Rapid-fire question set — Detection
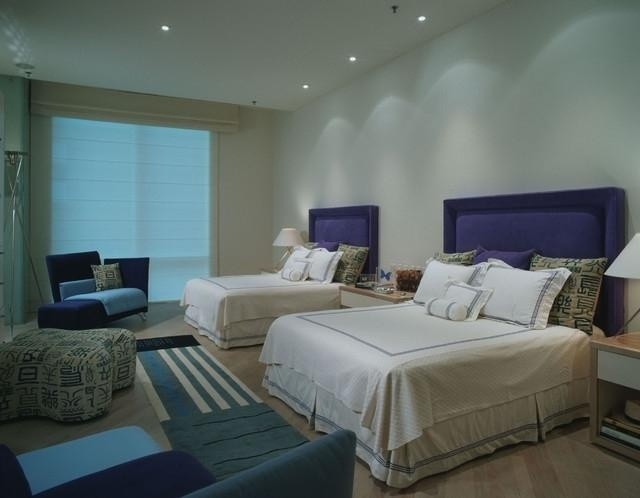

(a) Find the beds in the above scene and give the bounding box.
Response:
[271,187,625,489]
[186,205,379,350]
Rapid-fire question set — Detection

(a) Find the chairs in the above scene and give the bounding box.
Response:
[37,251,150,328]
[0,425,356,498]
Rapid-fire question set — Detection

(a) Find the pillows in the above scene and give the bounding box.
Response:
[90,262,124,292]
[278,240,370,285]
[412,247,609,336]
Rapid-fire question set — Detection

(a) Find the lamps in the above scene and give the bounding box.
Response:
[603,233,640,279]
[271,228,304,257]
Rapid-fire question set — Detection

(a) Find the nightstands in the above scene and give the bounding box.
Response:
[340,283,415,310]
[260,269,275,275]
[589,332,640,464]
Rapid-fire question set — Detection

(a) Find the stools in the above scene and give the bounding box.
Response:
[0,328,137,423]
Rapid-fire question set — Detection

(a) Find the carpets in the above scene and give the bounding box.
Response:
[136,335,312,484]
